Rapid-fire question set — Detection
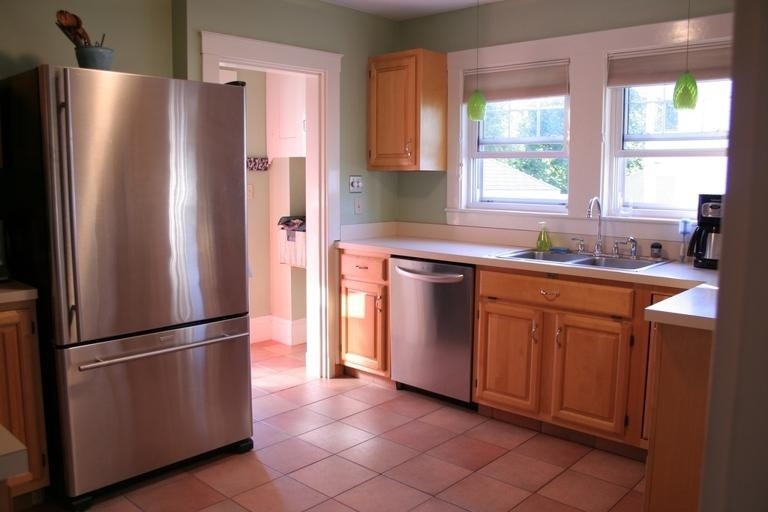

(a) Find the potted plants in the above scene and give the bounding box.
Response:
[52,9,115,71]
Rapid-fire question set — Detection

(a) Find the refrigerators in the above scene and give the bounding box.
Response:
[1,65,253,501]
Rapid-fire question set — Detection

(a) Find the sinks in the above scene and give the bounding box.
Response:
[573,252,674,273]
[497,248,587,268]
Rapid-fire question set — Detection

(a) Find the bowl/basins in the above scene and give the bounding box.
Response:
[73,47,115,73]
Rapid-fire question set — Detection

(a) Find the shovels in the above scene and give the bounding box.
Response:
[56,10,84,48]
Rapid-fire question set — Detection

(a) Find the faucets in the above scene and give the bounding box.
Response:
[628,237,638,260]
[586,196,604,258]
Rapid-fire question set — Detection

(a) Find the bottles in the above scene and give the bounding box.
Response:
[650,242,662,258]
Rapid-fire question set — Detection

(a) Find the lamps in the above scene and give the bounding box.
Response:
[670,0,700,110]
[465,0,489,121]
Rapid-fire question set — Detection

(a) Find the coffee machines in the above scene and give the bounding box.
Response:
[686,194,725,270]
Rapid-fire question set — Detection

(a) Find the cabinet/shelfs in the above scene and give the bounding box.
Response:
[0,298,51,511]
[625,282,678,450]
[364,45,444,173]
[337,249,397,390]
[470,264,635,457]
[265,69,307,157]
[641,321,713,512]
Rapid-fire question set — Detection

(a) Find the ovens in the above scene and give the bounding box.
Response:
[387,256,477,412]
